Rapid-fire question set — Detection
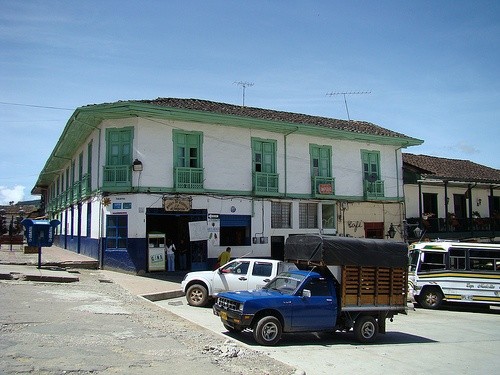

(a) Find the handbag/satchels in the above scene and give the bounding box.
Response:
[172,244,176,252]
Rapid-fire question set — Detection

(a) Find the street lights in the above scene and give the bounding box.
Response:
[389,223,405,239]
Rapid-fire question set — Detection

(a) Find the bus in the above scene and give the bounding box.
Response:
[403,242,499,308]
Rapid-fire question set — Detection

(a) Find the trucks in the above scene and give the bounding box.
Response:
[213,234,413,345]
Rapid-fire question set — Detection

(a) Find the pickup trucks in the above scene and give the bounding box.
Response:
[181,257,282,308]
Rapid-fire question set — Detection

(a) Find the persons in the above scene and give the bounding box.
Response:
[165,239,176,272]
[177,239,188,270]
[218,247,231,266]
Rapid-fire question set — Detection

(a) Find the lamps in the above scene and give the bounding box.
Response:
[388,222,401,239]
[413,227,423,238]
[133,158,143,171]
